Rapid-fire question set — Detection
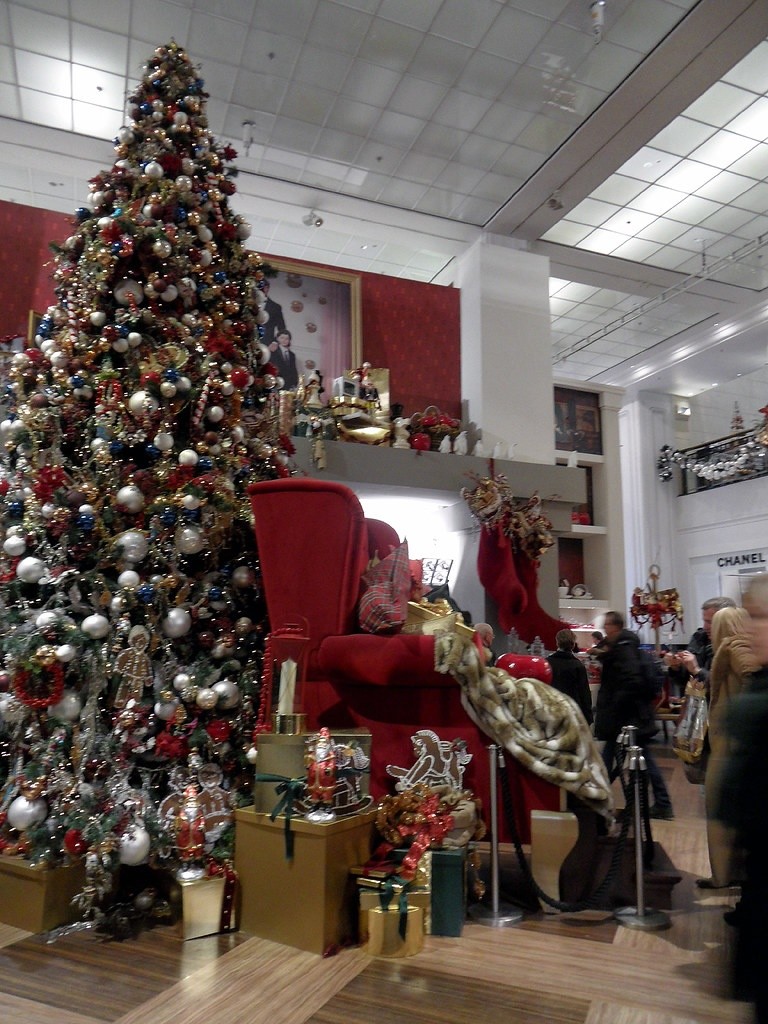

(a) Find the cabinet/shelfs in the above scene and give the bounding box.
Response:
[554,446,615,608]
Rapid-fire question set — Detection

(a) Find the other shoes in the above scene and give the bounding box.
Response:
[648,803,674,820]
[695,878,731,890]
[613,809,635,823]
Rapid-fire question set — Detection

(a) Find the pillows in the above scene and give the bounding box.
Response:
[400,612,458,634]
[356,536,411,634]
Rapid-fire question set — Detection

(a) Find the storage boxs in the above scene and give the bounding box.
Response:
[350,810,578,958]
[0,852,86,933]
[578,651,604,683]
[405,600,476,643]
[256,729,372,814]
[233,804,381,957]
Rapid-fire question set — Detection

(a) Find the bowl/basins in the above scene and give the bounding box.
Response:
[558,587,568,596]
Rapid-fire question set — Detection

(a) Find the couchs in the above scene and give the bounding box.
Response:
[245,480,559,860]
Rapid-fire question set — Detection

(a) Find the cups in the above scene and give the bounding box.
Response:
[275,714,307,736]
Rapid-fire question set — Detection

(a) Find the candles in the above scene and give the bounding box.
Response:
[278,658,296,713]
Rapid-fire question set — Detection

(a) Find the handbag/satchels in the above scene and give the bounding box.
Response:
[683,731,712,784]
[673,678,708,763]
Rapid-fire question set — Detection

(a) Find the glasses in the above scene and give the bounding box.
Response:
[486,631,496,638]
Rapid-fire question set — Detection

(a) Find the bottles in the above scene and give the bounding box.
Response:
[530,636,546,658]
[507,628,519,656]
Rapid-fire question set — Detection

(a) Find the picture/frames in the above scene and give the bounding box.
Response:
[256,255,362,407]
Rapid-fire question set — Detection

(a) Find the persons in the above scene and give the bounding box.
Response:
[176,789,205,871]
[260,280,299,391]
[545,629,594,727]
[306,727,336,813]
[588,612,675,824]
[473,623,497,667]
[664,573,768,1024]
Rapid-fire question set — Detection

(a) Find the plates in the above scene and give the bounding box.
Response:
[559,595,572,599]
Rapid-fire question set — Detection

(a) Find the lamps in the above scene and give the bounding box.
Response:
[302,207,323,227]
